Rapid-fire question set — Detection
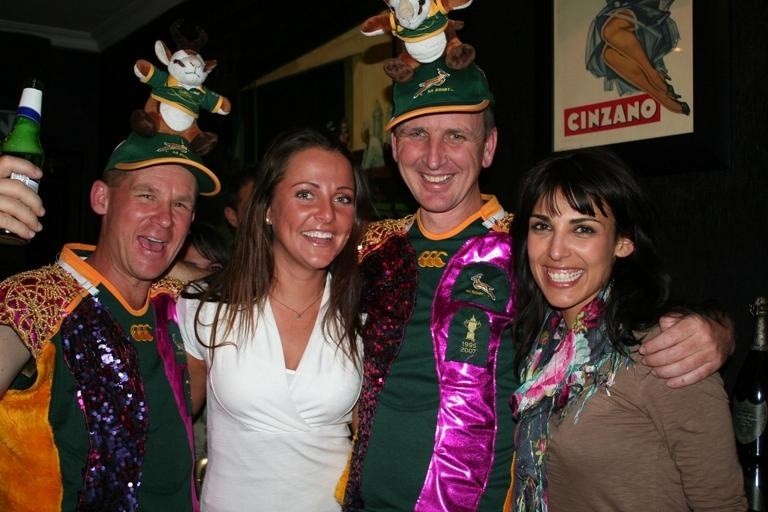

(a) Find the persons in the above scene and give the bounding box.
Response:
[0,130,204,512]
[198,151,258,230]
[166,50,739,511]
[582,1,692,115]
[503,145,752,511]
[174,125,367,512]
[177,207,238,273]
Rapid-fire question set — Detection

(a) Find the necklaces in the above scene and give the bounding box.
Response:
[265,283,325,323]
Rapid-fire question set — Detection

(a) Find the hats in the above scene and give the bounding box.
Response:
[386,58,492,130]
[102,133,221,198]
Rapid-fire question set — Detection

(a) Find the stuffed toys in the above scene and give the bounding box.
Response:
[359,0,480,85]
[128,39,235,160]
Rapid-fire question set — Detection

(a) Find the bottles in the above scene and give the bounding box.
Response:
[1,74,47,246]
[731,294,766,512]
